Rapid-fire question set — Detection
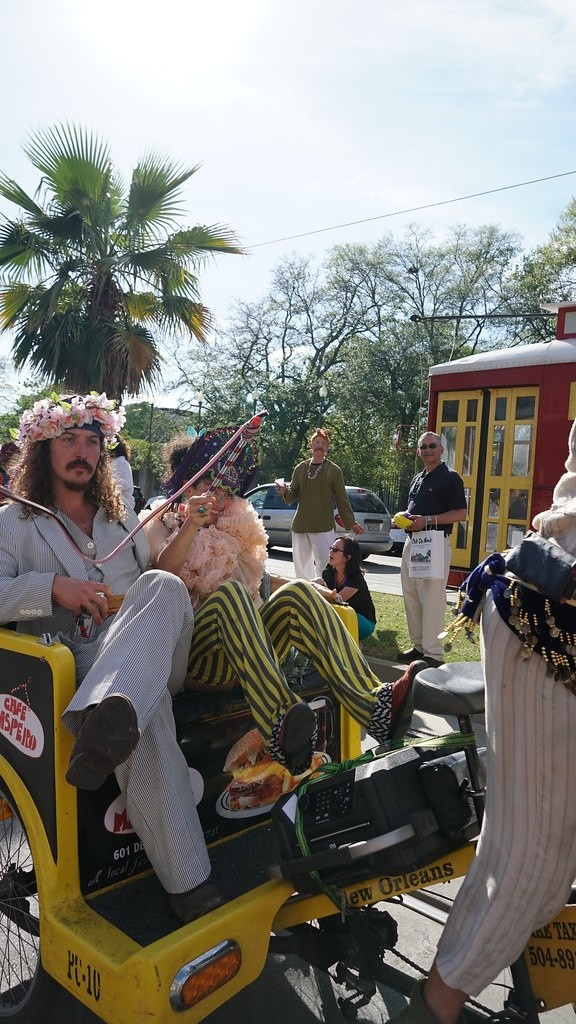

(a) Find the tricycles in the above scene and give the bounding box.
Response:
[0,570,576,1023]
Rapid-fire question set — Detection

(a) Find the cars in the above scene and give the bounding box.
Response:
[133,486,148,515]
[383,517,408,555]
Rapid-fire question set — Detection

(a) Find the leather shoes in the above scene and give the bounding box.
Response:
[65,692,140,792]
[168,878,228,924]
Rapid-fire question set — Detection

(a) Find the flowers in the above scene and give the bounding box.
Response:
[9,390,129,452]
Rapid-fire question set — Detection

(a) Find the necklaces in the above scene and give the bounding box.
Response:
[409,461,443,501]
[335,576,345,587]
[307,456,325,479]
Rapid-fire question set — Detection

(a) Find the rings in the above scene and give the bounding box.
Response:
[197,504,206,513]
[201,513,202,517]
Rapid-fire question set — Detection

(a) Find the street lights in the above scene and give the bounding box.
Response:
[196,393,205,439]
[147,393,156,444]
[316,384,329,429]
[252,387,259,416]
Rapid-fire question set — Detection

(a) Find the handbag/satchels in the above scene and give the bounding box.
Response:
[408,514,445,578]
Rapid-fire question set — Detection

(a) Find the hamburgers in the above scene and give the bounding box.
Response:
[223,726,324,809]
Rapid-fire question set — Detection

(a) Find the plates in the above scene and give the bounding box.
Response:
[216,750,332,819]
[104,767,204,835]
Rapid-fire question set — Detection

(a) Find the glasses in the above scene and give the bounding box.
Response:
[329,546,344,552]
[417,443,438,450]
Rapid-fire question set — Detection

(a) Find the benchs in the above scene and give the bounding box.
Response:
[0,580,366,899]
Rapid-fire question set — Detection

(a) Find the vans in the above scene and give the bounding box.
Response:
[244,482,394,561]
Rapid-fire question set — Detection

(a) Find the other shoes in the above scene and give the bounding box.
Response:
[387,659,429,740]
[397,647,424,660]
[420,656,445,669]
[278,702,317,776]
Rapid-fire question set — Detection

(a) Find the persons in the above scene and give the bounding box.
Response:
[392,432,467,668]
[0,390,230,927]
[138,437,431,775]
[322,537,377,639]
[274,432,364,580]
[106,433,135,510]
[0,441,21,506]
[384,417,576,1024]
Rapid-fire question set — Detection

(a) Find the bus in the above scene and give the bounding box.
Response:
[394,299,575,589]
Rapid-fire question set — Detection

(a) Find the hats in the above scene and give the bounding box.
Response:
[0,441,20,465]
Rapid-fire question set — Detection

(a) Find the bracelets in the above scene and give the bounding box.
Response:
[427,515,433,525]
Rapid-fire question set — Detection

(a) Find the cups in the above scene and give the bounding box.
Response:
[275,478,284,491]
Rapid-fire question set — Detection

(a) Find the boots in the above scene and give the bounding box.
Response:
[383,978,467,1024]
